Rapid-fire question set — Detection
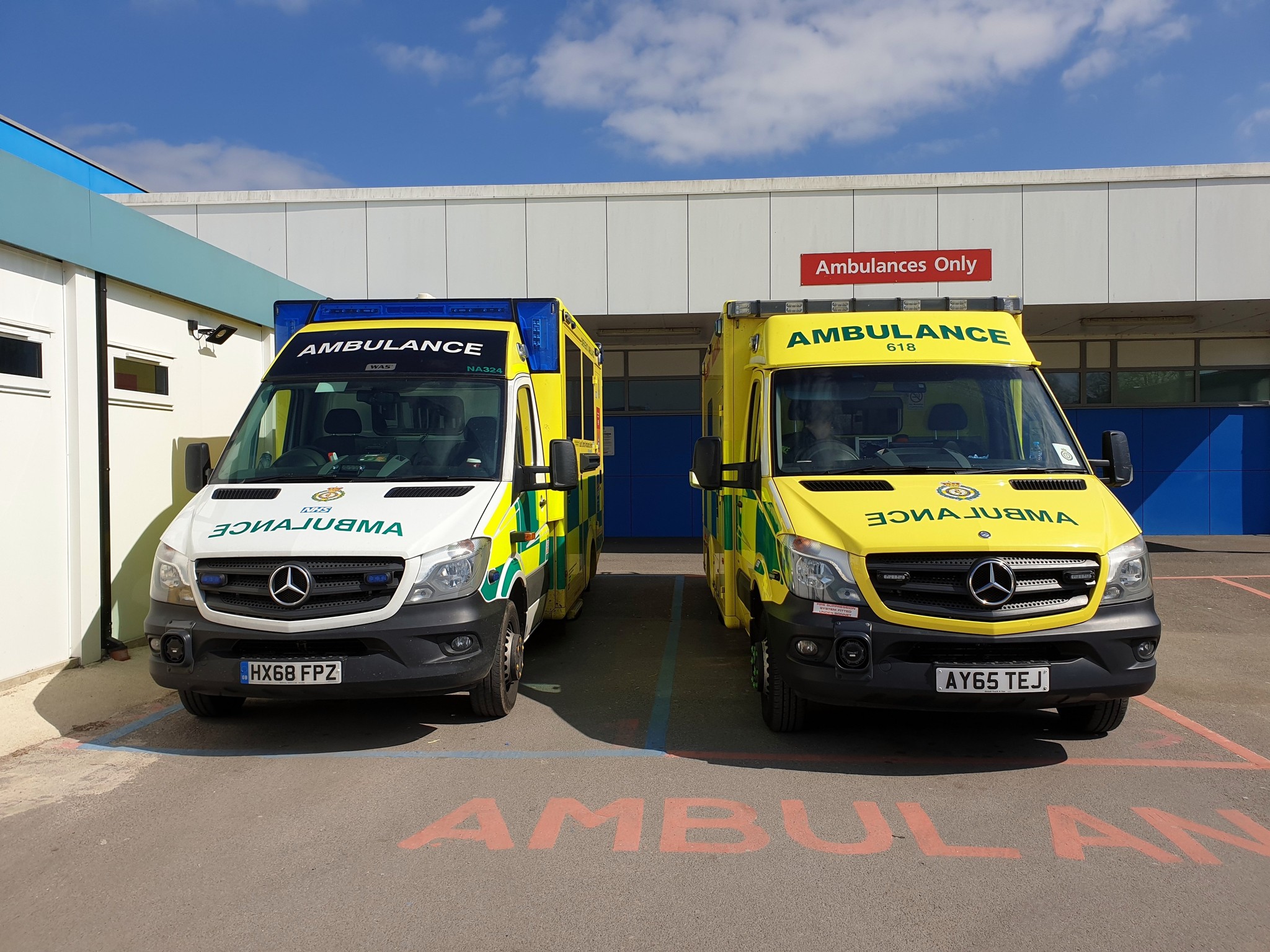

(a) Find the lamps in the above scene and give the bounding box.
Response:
[188,320,238,345]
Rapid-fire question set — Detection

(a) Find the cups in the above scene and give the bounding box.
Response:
[467,459,481,468]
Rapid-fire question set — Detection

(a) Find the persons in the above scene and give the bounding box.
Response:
[781,406,835,464]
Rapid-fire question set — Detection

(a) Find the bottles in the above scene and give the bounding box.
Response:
[257,451,272,467]
[1030,442,1042,461]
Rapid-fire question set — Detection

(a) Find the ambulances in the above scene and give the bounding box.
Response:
[142,291,607,724]
[687,297,1164,740]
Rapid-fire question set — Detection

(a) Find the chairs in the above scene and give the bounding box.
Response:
[788,398,829,434]
[312,408,366,456]
[447,416,498,467]
[929,403,968,440]
